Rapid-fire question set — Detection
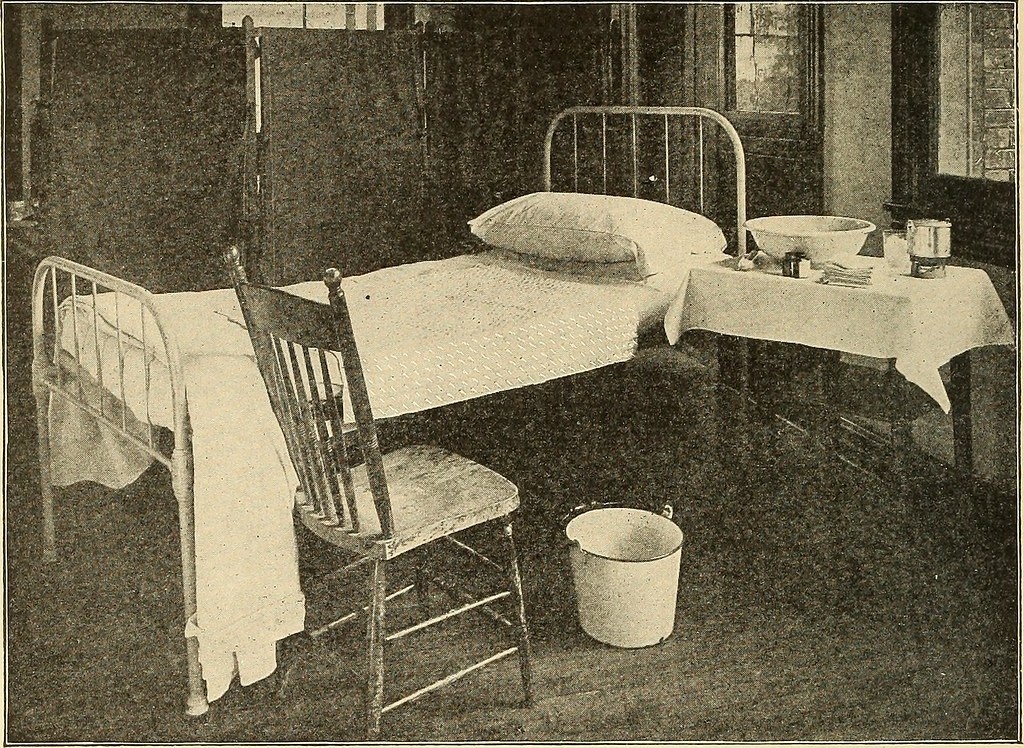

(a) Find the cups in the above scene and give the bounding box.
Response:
[882,229,911,275]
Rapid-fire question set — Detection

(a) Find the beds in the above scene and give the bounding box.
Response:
[31,106,747,717]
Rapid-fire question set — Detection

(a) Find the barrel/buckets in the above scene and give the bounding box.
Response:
[559,502,683,649]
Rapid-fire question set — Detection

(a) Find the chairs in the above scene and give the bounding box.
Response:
[219,244,538,742]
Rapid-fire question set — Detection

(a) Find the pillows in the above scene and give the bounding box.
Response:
[467,191,728,282]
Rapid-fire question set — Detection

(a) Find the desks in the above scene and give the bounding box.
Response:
[663,251,1018,597]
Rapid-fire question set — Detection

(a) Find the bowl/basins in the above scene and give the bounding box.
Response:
[743,215,875,263]
